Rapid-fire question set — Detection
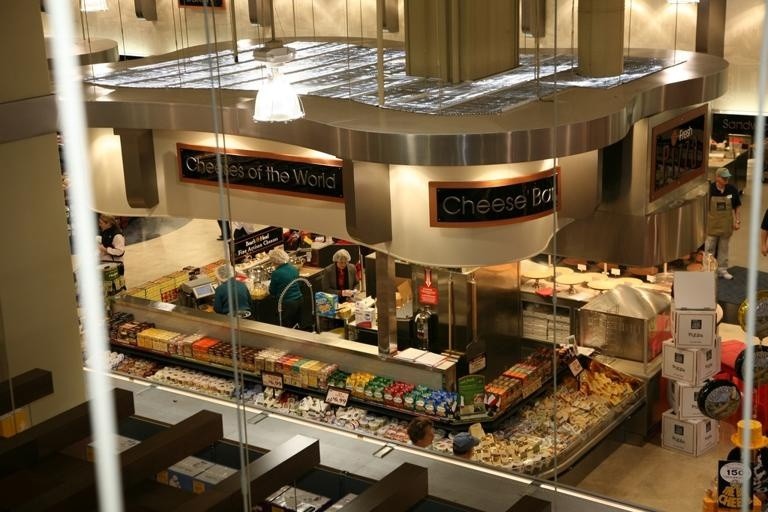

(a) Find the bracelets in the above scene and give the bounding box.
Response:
[735,221,741,224]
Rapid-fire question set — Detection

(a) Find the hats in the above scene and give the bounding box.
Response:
[452,432,481,455]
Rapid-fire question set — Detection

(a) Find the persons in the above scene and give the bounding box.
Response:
[233,222,255,241]
[704,167,743,281]
[760,207,768,257]
[266,249,305,328]
[216,219,231,242]
[452,432,482,459]
[404,416,437,451]
[282,229,300,250]
[320,248,361,303]
[213,264,253,315]
[96,214,126,265]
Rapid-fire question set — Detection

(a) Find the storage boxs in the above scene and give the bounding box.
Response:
[659,298,724,456]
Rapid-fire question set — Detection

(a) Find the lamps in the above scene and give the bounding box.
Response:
[252,1,306,124]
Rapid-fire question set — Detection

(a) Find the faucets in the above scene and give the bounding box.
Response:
[277,277,318,332]
